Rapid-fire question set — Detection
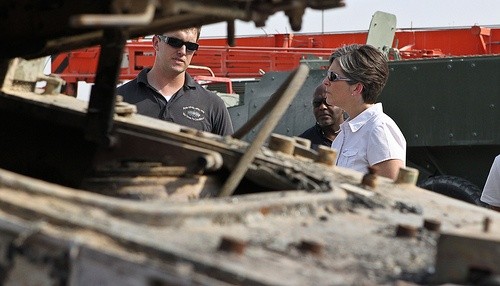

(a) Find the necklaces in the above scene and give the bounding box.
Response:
[334,127,341,134]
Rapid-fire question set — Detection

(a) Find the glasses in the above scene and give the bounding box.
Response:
[312,98,331,107]
[326,70,352,82]
[156,34,199,51]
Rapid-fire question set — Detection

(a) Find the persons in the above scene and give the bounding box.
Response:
[295,83,348,149]
[116,25,234,138]
[480,155,500,213]
[323,43,407,181]
